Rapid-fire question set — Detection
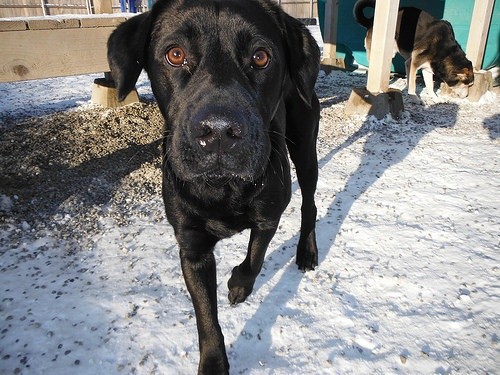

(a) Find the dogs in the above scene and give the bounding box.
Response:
[352,0,475,98]
[107,0,322,375]
